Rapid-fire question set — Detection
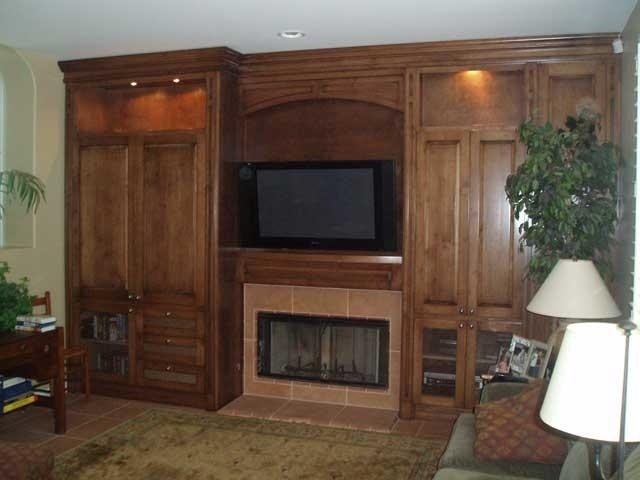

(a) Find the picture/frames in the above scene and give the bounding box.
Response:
[509,333,535,375]
[524,339,554,381]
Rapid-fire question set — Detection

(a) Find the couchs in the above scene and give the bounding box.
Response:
[432,383,590,480]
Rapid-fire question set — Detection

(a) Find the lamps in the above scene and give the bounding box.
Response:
[540,321,640,468]
[526,258,622,320]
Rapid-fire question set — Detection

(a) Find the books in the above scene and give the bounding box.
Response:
[90,311,127,343]
[15,313,56,333]
[96,349,129,378]
[0,360,70,414]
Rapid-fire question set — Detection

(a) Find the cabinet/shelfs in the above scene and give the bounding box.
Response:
[397,31,618,426]
[57,46,241,414]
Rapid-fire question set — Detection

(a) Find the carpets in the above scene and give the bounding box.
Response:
[52,407,447,480]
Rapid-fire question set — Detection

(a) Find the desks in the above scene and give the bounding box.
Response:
[0,327,66,434]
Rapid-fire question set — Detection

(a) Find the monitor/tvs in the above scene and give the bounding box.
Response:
[237,159,397,252]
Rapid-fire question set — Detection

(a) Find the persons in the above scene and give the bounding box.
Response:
[498,344,548,379]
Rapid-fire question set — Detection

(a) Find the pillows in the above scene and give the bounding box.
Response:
[473,383,568,464]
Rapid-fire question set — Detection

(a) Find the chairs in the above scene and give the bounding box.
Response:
[25,291,90,401]
